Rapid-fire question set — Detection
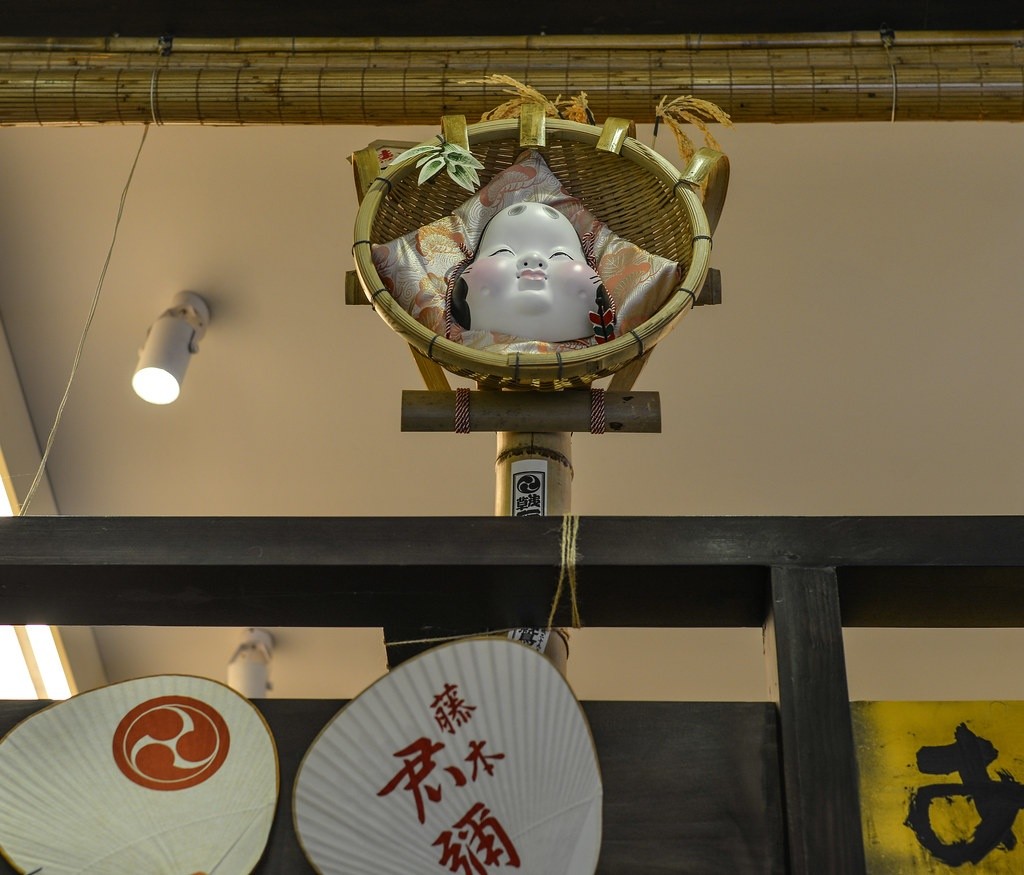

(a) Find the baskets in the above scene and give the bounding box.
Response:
[352,117,713,392]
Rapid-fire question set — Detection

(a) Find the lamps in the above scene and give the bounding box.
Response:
[228,628,277,699]
[131,293,215,405]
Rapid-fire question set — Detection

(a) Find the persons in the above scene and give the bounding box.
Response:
[451,202,617,344]
